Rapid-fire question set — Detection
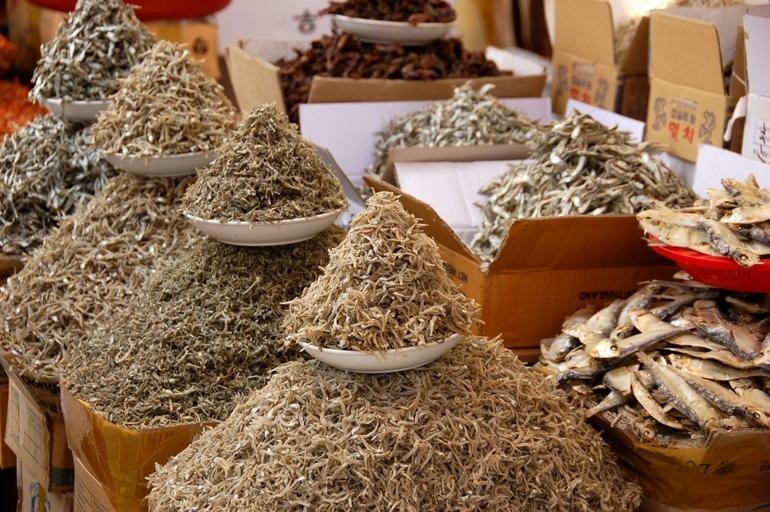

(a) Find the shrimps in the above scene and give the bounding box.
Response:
[0,0,770,512]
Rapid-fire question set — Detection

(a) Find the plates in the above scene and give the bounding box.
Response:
[297,333,465,376]
[186,208,346,248]
[329,12,457,48]
[642,222,770,293]
[103,146,222,178]
[42,96,115,121]
